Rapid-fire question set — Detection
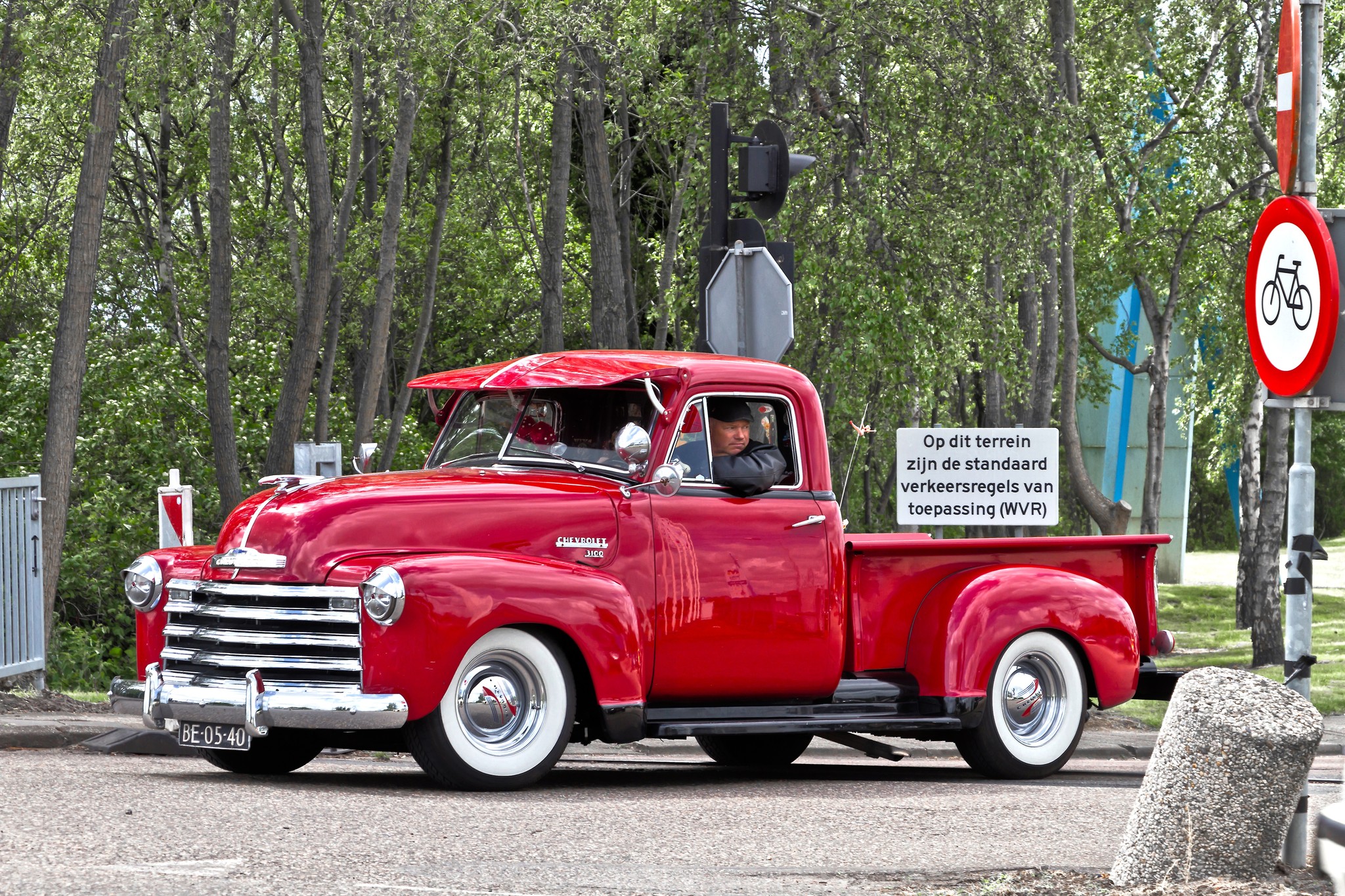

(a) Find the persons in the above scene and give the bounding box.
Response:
[670,397,789,496]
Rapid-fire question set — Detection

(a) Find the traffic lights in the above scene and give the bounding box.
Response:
[738,119,816,219]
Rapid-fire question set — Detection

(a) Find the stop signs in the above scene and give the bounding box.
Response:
[1277,0,1303,194]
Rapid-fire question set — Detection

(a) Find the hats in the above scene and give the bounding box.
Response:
[707,396,754,422]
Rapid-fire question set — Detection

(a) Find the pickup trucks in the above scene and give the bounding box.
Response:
[119,348,1179,796]
